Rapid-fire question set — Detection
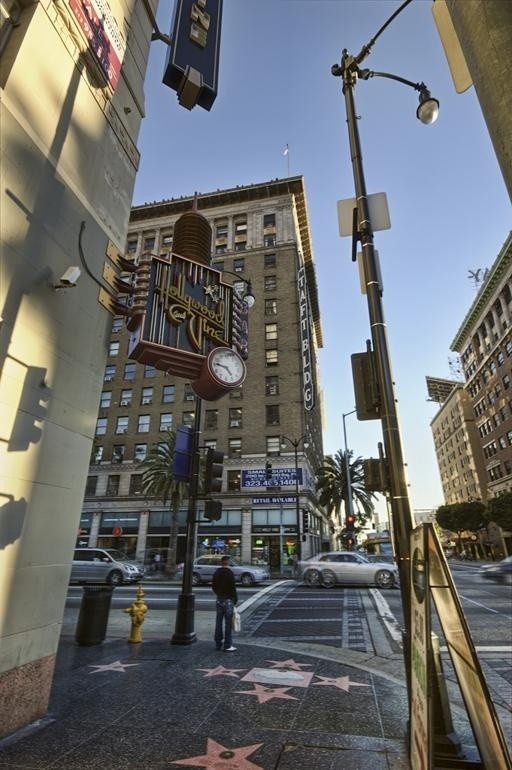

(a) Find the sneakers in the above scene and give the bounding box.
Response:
[224,645,238,653]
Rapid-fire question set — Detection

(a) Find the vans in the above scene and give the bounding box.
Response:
[134,547,176,569]
[66,546,150,586]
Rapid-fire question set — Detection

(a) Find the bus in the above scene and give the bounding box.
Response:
[361,531,394,556]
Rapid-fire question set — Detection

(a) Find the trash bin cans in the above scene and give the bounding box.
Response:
[75,586,115,646]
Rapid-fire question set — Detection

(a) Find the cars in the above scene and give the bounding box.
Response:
[366,551,395,564]
[174,552,274,587]
[476,556,512,587]
[292,550,399,590]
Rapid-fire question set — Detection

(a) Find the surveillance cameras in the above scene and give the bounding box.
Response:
[59,263,82,288]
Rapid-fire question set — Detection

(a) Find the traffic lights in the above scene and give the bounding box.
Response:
[346,532,356,546]
[346,514,355,531]
[265,460,273,481]
[303,508,310,533]
[204,444,226,494]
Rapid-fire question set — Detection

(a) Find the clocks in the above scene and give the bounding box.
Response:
[188,342,250,403]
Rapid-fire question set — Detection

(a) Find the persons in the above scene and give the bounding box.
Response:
[212,556,238,651]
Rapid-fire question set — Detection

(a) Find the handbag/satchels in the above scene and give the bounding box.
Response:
[232,606,241,633]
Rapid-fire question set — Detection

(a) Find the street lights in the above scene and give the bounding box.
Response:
[324,0,442,767]
[278,432,312,562]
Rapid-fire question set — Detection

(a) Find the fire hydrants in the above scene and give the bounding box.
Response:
[120,584,149,642]
